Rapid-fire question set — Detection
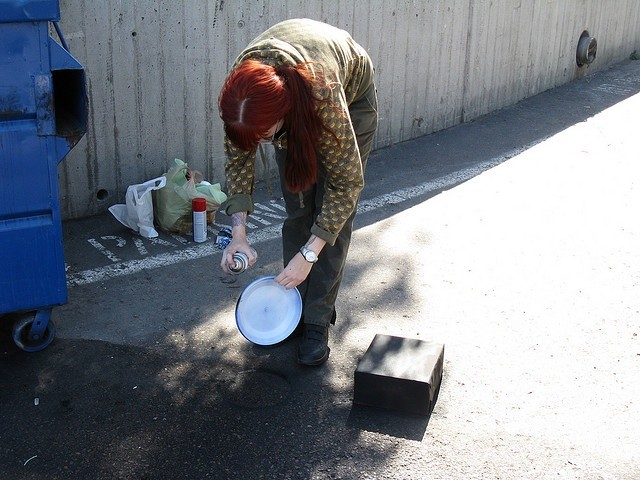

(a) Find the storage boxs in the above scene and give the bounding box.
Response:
[353,334,444,417]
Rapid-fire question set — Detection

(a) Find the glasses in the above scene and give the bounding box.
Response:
[257,124,279,145]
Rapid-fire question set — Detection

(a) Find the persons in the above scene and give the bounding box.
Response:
[218,18,379,367]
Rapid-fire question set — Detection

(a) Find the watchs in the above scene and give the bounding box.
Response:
[299,246,319,264]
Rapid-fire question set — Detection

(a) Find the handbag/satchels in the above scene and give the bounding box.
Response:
[108,175,167,238]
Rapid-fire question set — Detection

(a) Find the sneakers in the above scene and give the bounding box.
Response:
[296,320,330,366]
